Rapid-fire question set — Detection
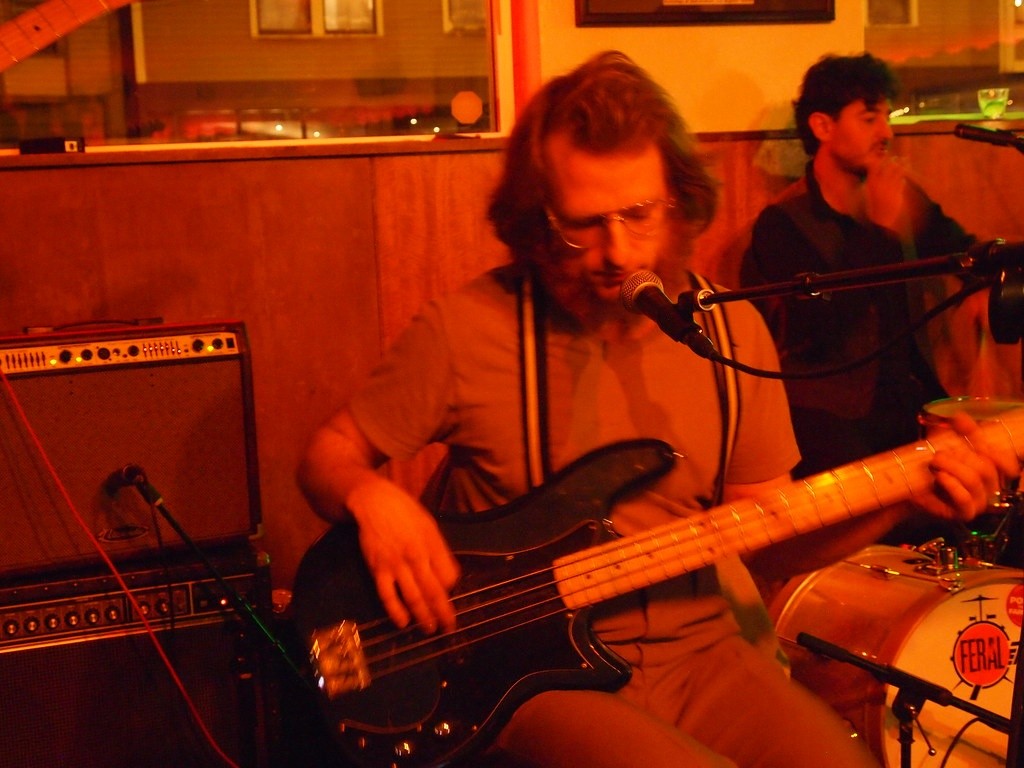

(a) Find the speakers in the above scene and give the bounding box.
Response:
[0,321,263,582]
[0,555,293,768]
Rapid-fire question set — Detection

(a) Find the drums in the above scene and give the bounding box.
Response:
[772,540,1024,767]
[918,392,1024,517]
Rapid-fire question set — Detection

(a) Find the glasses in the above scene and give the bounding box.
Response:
[539,189,678,250]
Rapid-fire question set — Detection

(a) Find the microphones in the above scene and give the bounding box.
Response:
[954,123,1024,151]
[122,463,164,508]
[620,268,719,361]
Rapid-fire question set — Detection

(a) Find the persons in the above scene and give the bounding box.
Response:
[733,50,1022,548]
[295,56,1020,768]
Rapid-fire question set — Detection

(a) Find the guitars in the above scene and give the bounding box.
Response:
[286,403,1024,768]
